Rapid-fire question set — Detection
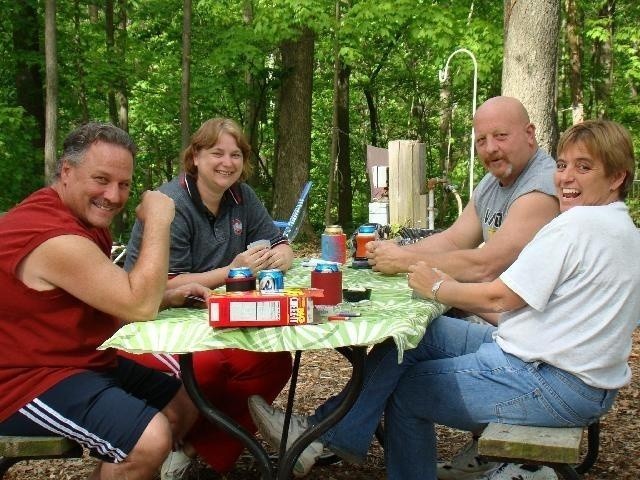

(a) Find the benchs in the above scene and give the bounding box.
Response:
[477,423,601,480]
[3,434,85,480]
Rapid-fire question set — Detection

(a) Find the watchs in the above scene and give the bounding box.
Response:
[431,278,445,303]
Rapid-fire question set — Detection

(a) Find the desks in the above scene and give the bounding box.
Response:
[94,242,450,478]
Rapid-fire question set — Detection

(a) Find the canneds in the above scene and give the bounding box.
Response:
[228,266,252,279]
[359,226,377,233]
[324,225,344,235]
[315,262,339,273]
[258,268,283,292]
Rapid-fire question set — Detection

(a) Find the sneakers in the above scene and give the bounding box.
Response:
[436,440,558,480]
[248,395,323,477]
[161,448,191,480]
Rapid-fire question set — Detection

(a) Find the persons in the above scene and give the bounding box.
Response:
[246,114,640,480]
[368,96,563,290]
[122,116,298,480]
[0,123,215,480]
